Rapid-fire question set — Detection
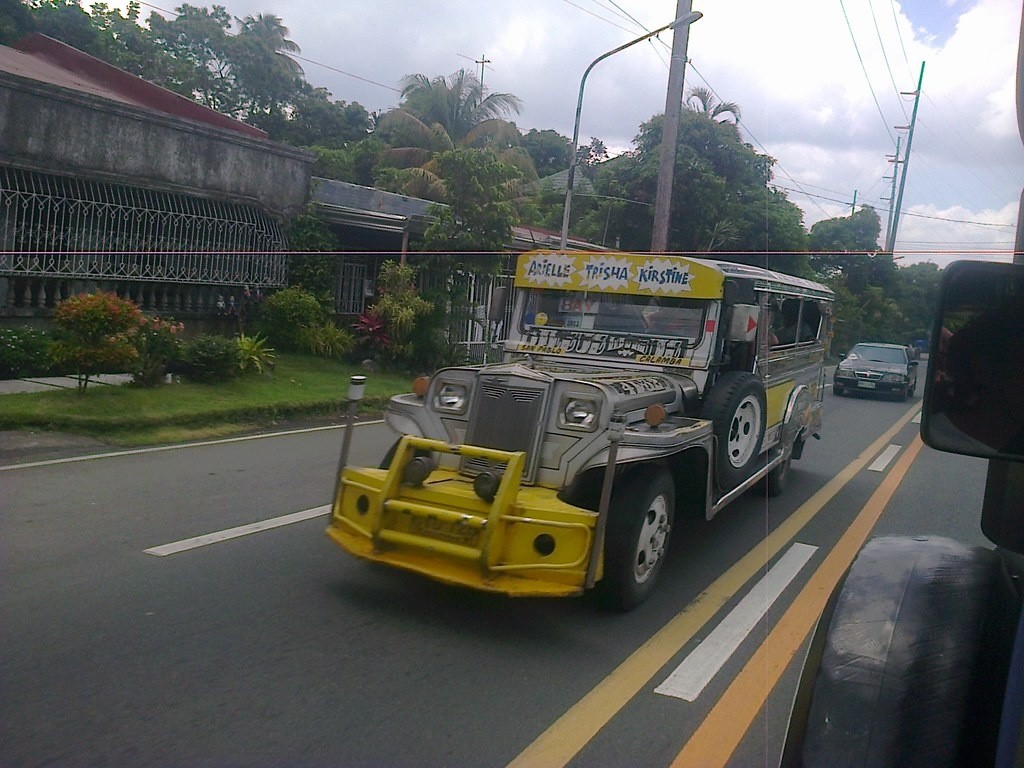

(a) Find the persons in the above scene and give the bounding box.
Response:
[775,300,815,343]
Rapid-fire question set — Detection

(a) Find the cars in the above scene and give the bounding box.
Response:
[831,343,918,402]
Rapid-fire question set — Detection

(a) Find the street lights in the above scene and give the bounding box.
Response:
[559,10,703,249]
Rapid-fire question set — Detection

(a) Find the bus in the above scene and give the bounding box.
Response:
[323,252,836,611]
